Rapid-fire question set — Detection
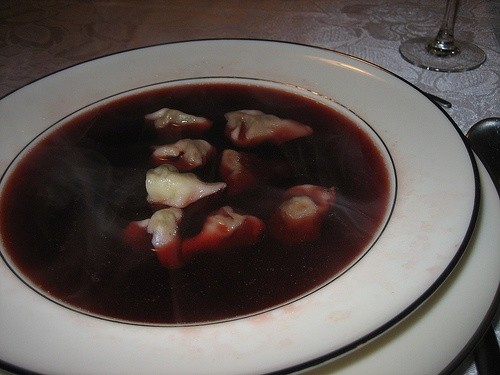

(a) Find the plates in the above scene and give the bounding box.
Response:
[300,137,500,375]
[0,39,481,375]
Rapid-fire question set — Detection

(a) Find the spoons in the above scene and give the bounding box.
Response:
[466,117,500,197]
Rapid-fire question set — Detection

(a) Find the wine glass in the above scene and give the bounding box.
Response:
[399,0,488,72]
